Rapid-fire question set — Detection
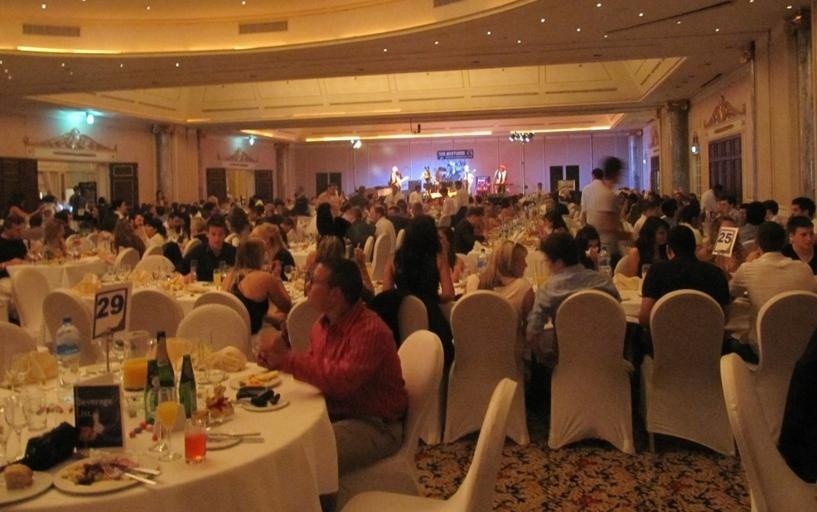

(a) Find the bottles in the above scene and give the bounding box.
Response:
[175,354,198,418]
[54,316,81,403]
[475,248,486,272]
[146,331,174,424]
[598,247,612,278]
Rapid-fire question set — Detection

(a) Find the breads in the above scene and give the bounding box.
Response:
[4,464,32,491]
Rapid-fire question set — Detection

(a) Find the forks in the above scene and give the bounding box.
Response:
[101,463,156,487]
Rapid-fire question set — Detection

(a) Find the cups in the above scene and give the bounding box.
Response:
[641,264,650,278]
[486,212,530,248]
[121,332,149,391]
[182,414,206,466]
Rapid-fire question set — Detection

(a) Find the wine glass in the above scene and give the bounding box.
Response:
[202,354,227,395]
[93,330,126,378]
[77,260,315,303]
[142,383,182,466]
[0,352,48,453]
[28,239,108,265]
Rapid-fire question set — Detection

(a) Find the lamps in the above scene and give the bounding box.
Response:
[348,138,362,150]
[508,128,534,144]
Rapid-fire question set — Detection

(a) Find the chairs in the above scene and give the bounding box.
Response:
[639,287,739,463]
[341,375,522,512]
[545,288,635,455]
[333,326,442,510]
[718,351,815,511]
[744,288,817,445]
[0,226,445,377]
[441,289,530,447]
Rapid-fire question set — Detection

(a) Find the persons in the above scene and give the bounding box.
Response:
[1,154,816,483]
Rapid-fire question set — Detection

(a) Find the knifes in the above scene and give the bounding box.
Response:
[208,432,262,446]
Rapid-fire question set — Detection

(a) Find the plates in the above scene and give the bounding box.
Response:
[202,430,239,449]
[229,372,290,413]
[0,466,53,506]
[55,458,153,493]
